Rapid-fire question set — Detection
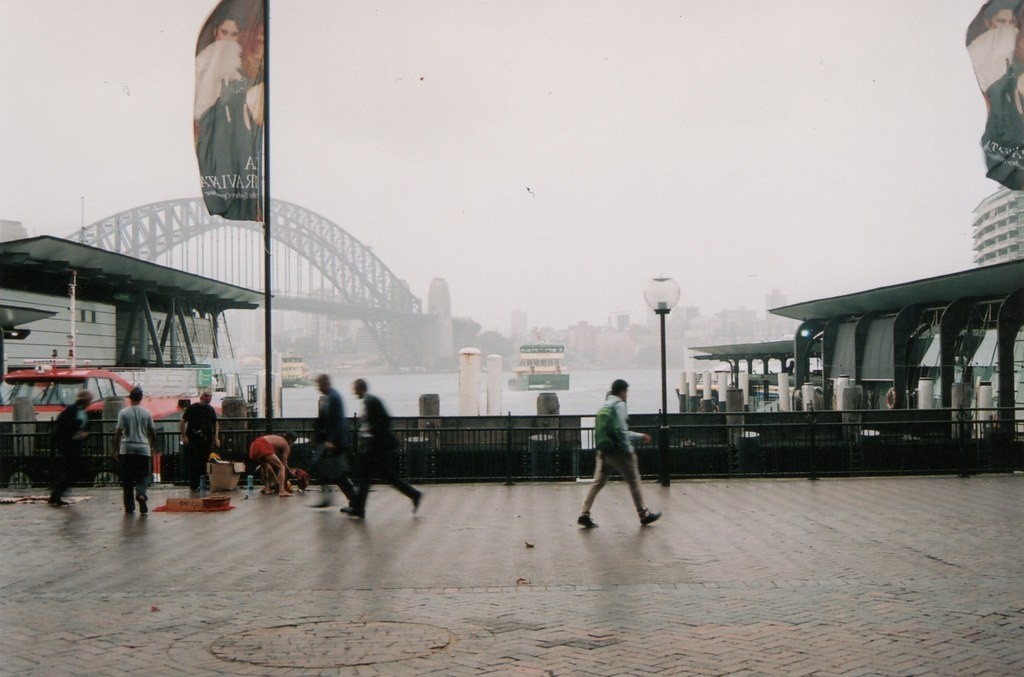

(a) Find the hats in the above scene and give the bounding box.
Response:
[75,389,94,401]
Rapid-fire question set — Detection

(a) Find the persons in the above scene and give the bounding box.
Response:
[577,379,661,528]
[48,390,98,506]
[181,389,222,497]
[113,387,157,514]
[968,3,1023,188]
[194,14,259,216]
[249,374,423,520]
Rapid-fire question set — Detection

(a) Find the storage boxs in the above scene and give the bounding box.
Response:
[206,460,246,490]
[203,496,231,508]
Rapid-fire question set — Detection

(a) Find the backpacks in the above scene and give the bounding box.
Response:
[595,400,627,455]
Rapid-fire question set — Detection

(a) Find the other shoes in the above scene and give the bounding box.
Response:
[340,505,355,514]
[311,499,331,509]
[641,511,661,524]
[342,511,365,519]
[48,497,69,507]
[125,508,132,513]
[578,516,599,528]
[412,491,422,510]
[138,495,148,513]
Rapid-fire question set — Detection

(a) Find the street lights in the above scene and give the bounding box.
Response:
[643,274,681,487]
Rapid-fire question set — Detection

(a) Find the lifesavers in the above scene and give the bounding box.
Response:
[886,388,896,409]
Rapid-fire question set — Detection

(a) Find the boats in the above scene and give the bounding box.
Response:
[282,348,311,388]
[507,331,571,392]
[0,266,289,489]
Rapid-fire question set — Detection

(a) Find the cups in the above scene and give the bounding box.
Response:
[248,475,253,489]
[200,475,205,490]
